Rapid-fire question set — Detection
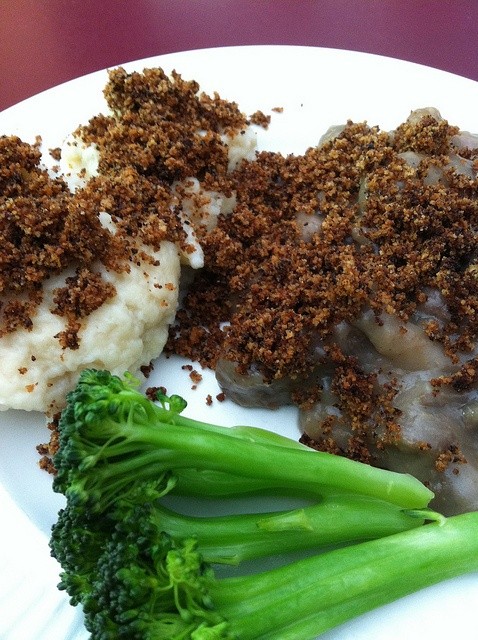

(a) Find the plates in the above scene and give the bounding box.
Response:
[0,43,478,640]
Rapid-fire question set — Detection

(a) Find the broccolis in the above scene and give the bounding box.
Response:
[35,371,477,639]
